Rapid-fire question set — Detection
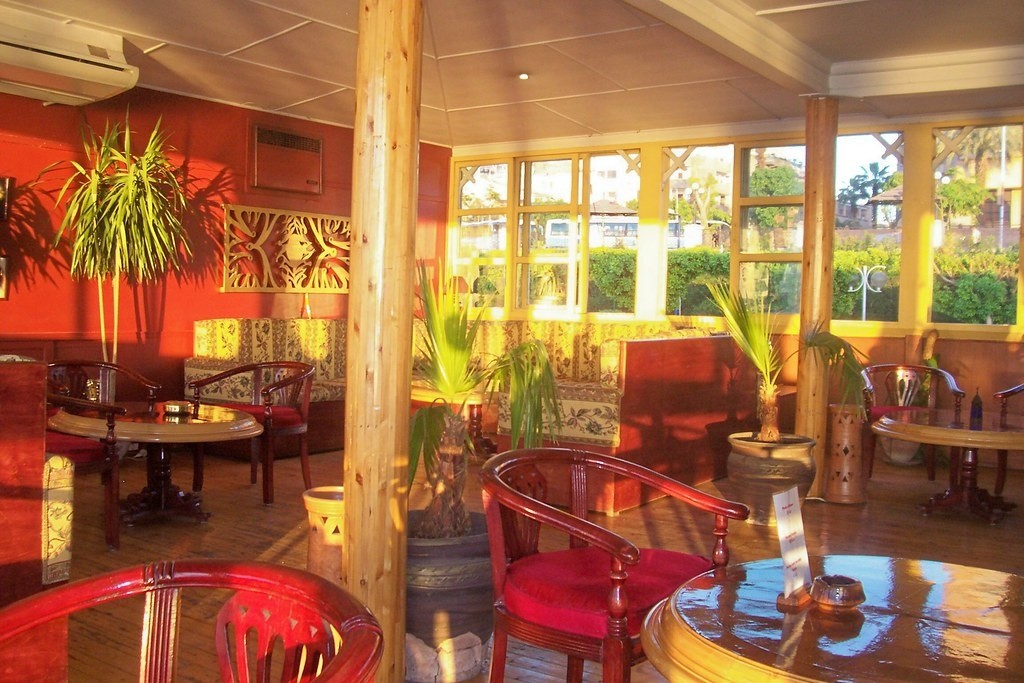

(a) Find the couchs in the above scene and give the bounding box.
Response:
[184,317,761,516]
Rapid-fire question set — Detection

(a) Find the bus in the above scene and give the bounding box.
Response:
[545,216,680,251]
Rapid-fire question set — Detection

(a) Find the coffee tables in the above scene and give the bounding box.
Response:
[411,380,502,455]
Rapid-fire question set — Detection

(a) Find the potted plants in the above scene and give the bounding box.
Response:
[702,279,874,527]
[406,257,564,683]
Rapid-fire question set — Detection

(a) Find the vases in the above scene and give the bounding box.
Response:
[302,486,345,586]
[824,402,865,505]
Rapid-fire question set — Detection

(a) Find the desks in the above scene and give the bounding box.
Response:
[48,400,265,525]
[871,409,1024,526]
[640,553,1024,683]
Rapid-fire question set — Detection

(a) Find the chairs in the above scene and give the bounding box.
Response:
[992,384,1024,495]
[0,558,385,682]
[477,448,753,683]
[861,363,966,498]
[46,358,317,553]
